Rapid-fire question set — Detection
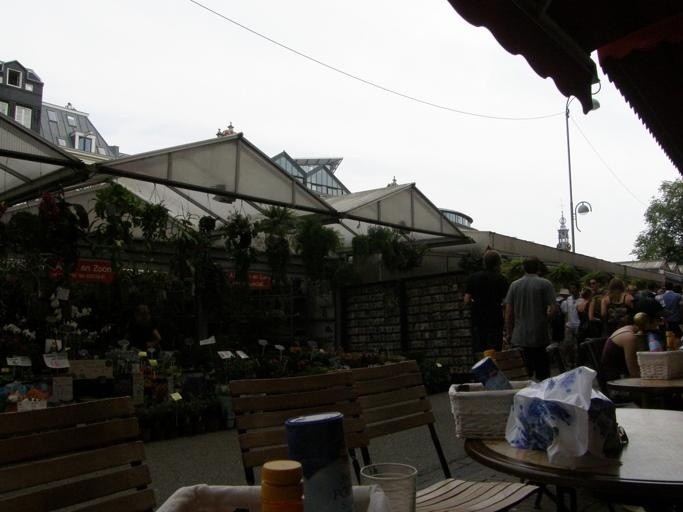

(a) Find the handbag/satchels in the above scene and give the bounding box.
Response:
[588,389,622,459]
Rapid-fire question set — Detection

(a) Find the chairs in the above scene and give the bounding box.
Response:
[583,334,635,409]
[486,347,531,381]
[0,395,158,511]
[349,360,540,512]
[230,369,370,485]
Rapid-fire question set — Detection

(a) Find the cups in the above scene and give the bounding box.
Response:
[360,462,418,512]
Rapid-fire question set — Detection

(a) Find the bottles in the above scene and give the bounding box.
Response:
[648,331,676,352]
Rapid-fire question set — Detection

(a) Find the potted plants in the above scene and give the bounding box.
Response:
[0,178,421,280]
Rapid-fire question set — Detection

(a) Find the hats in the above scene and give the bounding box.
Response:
[559,289,571,295]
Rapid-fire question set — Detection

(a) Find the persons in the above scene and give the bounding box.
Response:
[463,249,508,357]
[122,304,161,349]
[552,273,683,402]
[501,254,556,382]
